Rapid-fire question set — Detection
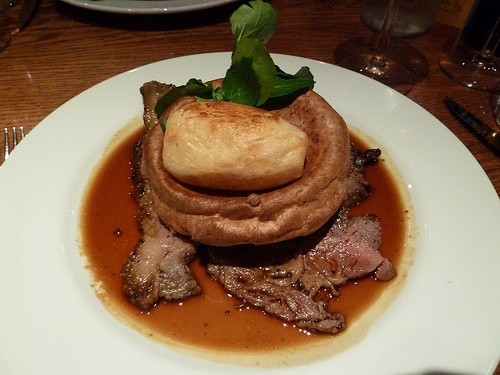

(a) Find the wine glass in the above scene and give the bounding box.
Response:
[333,0,422,96]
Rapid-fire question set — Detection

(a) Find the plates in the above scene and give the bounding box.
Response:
[65,0,229,15]
[0,49,500,374]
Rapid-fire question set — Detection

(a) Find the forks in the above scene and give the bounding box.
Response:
[3,125,24,162]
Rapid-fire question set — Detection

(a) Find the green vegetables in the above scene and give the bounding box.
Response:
[153,0,316,129]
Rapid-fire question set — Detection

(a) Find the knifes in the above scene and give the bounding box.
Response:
[15,0,37,31]
[445,96,500,151]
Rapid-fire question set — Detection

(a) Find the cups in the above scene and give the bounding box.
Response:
[440,0,500,92]
[360,0,439,38]
